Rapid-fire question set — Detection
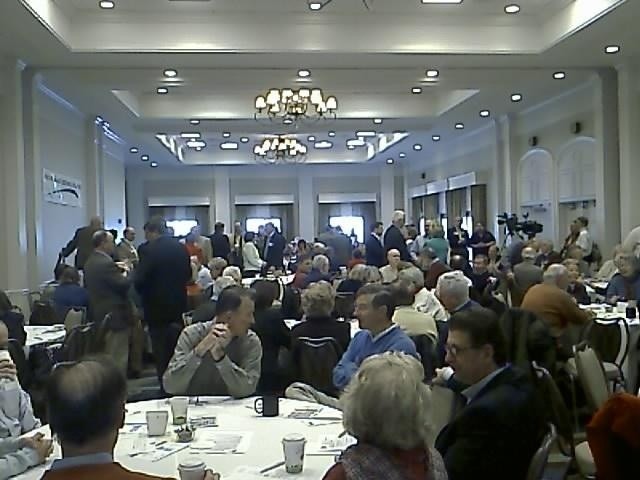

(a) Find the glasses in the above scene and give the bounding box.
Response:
[443,344,486,356]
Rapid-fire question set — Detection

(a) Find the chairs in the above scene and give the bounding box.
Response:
[64,306,85,344]
[625,325,639,395]
[566,317,628,398]
[182,310,196,326]
[100,310,112,353]
[204,284,213,300]
[57,324,95,363]
[531,359,574,434]
[291,336,342,399]
[573,340,610,412]
[28,300,54,324]
[334,290,356,320]
[526,422,561,479]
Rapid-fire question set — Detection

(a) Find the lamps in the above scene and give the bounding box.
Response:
[254,133,308,163]
[254,88,337,129]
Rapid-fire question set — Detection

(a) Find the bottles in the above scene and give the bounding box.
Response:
[626,300,637,319]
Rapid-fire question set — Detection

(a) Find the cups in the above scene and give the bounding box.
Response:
[170,396,189,425]
[282,432,307,474]
[617,301,626,313]
[177,457,207,480]
[146,411,169,437]
[254,391,280,416]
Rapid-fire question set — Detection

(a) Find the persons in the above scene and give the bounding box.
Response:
[28,210,639,411]
[0,319,41,438]
[435,307,548,479]
[0,432,54,480]
[0,289,27,346]
[322,352,450,479]
[40,350,220,480]
